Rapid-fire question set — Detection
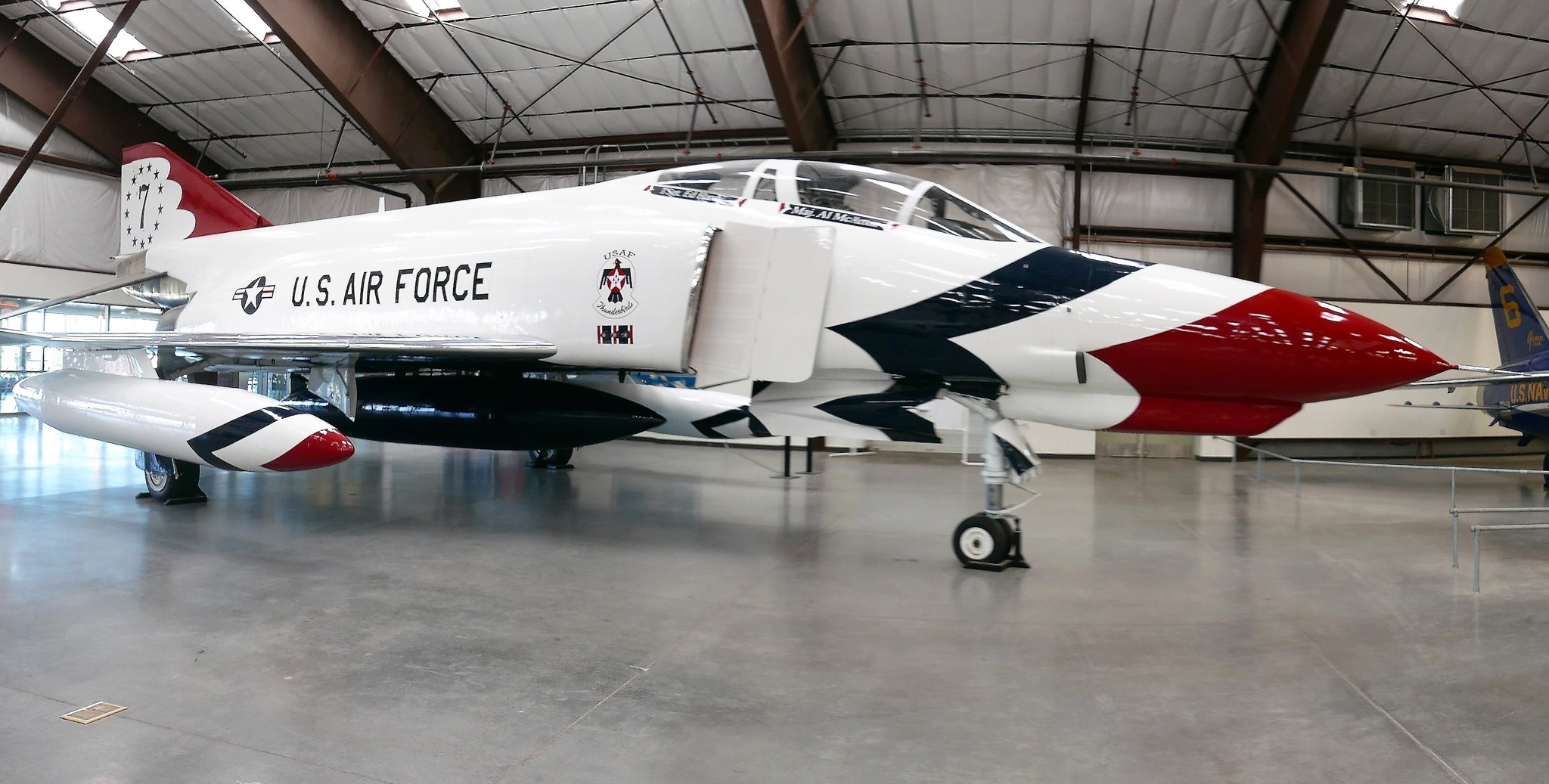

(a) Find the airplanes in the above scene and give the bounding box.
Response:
[1386,244,1549,491]
[1,141,1532,572]
